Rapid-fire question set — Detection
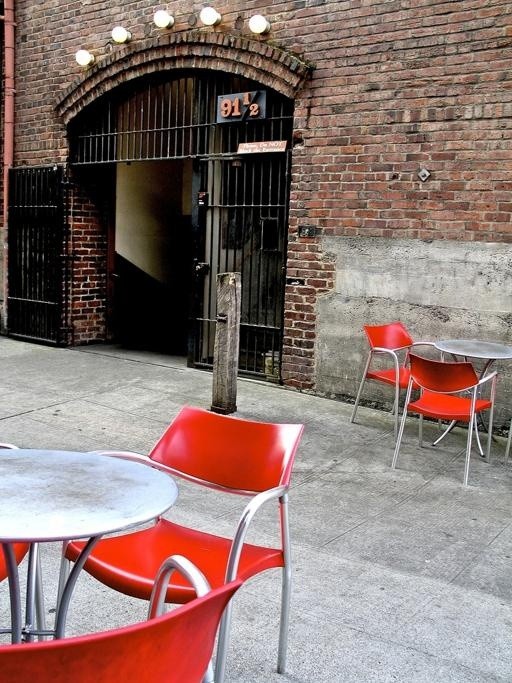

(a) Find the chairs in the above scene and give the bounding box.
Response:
[0,442,48,645]
[350,322,442,444]
[391,352,498,486]
[0,581,242,683]
[58,404,304,683]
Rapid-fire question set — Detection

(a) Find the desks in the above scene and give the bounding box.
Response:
[0,447,180,644]
[435,339,512,457]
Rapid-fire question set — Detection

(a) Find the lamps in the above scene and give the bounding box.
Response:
[248,15,271,34]
[112,26,132,42]
[154,10,175,29]
[75,49,94,67]
[199,7,221,26]
